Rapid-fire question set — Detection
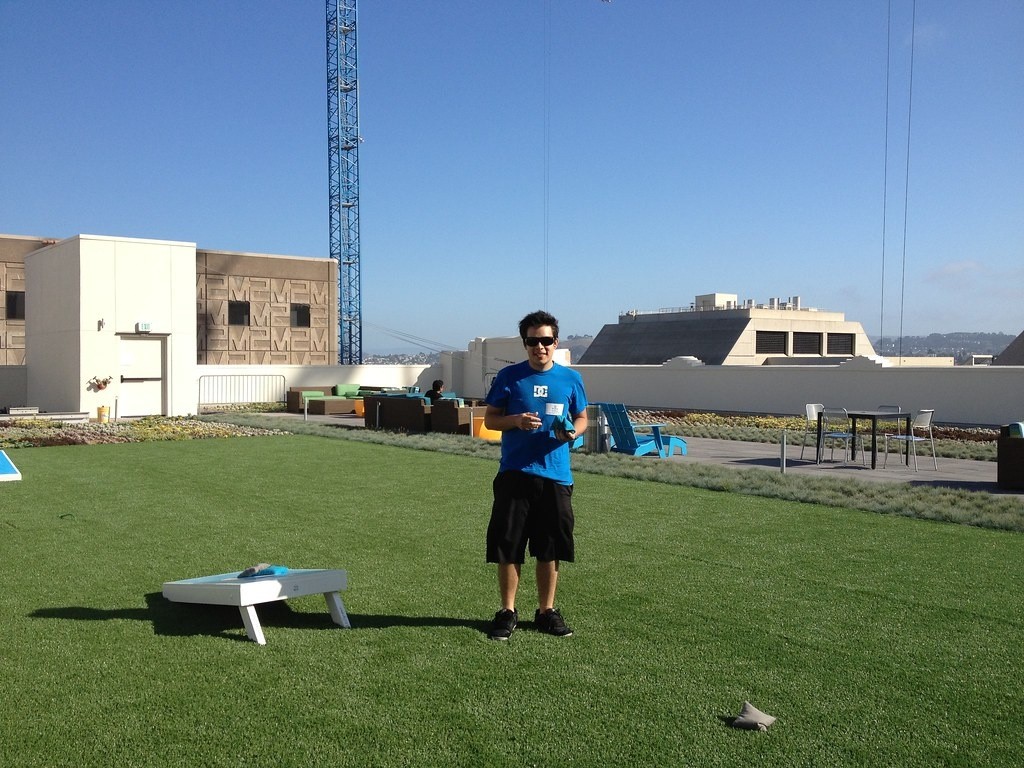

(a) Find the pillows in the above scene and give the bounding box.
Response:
[301,390,325,404]
[336,384,361,396]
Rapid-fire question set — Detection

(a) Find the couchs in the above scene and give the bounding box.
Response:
[286,384,383,414]
[433,397,488,435]
[363,392,435,435]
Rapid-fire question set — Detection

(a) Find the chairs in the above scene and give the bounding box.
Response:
[860,405,903,469]
[820,404,866,468]
[884,409,938,472]
[800,404,838,463]
[601,402,688,459]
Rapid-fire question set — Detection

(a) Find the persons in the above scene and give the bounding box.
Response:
[424,379,444,405]
[484,311,589,641]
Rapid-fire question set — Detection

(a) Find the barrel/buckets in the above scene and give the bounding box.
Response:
[583,404,611,453]
[355,400,365,417]
[997,436,1024,490]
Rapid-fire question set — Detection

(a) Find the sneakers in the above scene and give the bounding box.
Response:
[490,607,518,640]
[535,608,573,637]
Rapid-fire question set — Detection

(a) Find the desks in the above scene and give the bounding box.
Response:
[819,411,911,471]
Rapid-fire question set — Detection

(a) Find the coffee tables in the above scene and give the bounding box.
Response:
[309,399,357,415]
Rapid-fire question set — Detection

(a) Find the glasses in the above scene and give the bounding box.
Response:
[525,336,556,347]
